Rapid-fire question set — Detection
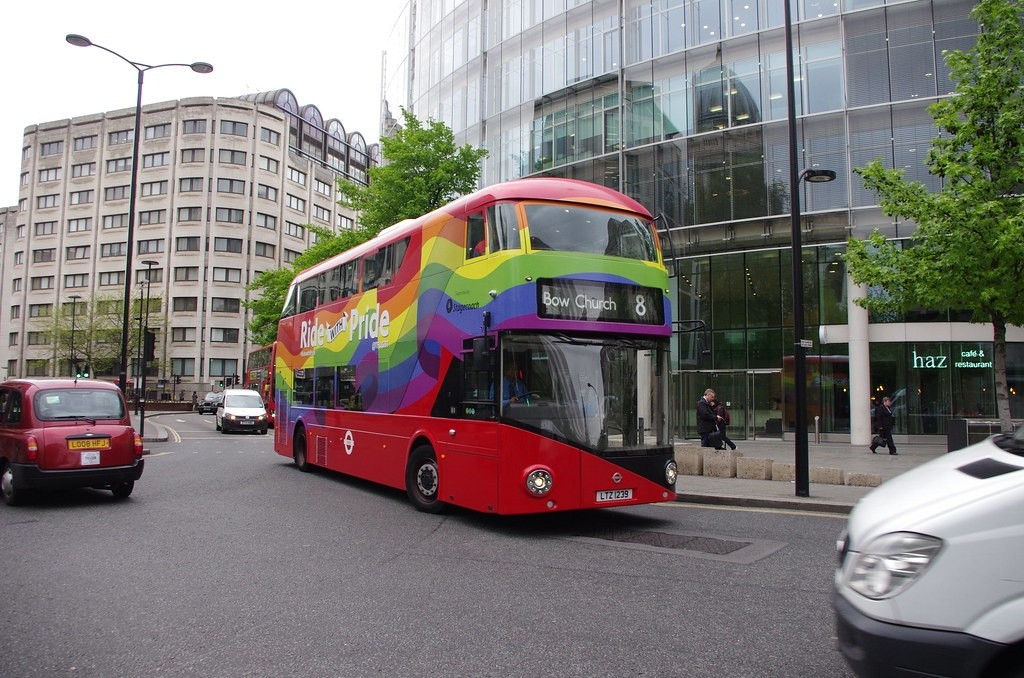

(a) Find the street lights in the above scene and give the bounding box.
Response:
[66,33,214,393]
[139,260,159,436]
[68,295,82,376]
[134,280,147,415]
[783,0,837,497]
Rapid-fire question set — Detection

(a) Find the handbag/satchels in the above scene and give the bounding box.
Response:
[872,436,887,448]
[708,430,722,444]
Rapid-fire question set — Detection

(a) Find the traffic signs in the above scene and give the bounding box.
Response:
[219,381,223,387]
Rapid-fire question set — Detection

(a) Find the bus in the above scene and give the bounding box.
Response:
[274,178,678,515]
[244,341,277,429]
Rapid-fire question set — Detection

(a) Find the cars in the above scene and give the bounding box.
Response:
[0,378,144,507]
[833,420,1023,678]
[197,391,223,415]
[216,389,269,434]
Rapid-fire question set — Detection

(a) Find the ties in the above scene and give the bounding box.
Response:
[508,383,516,399]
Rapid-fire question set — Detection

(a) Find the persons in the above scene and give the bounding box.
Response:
[711,398,737,450]
[344,393,357,410]
[696,389,724,450]
[870,397,899,455]
[489,359,533,409]
